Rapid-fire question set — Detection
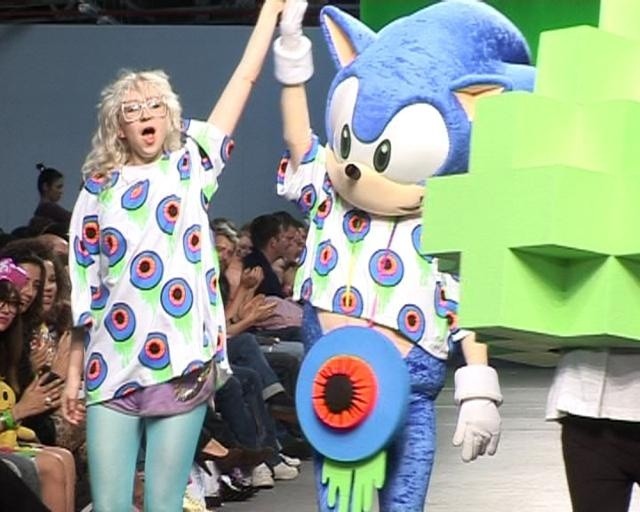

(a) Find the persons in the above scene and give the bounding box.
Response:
[2,163,309,512]
[60,0,287,512]
[544,348,640,512]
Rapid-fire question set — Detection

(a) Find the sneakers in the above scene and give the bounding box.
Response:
[279,454,301,466]
[271,462,299,480]
[205,479,250,508]
[251,464,276,492]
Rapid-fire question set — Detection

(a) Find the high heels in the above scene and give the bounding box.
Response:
[195,446,244,474]
[241,447,273,466]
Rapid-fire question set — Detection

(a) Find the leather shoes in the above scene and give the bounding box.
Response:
[269,403,298,426]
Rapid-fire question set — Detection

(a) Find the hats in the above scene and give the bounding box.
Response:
[0,259,29,289]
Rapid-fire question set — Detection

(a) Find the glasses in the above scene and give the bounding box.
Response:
[0,299,24,317]
[119,99,168,123]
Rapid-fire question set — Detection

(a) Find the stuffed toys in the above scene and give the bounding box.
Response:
[272,0,537,512]
